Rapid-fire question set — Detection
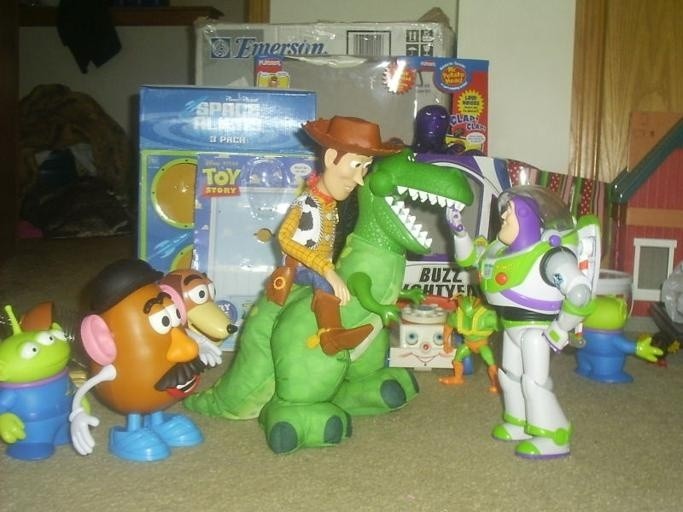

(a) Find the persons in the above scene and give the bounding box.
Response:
[278,113,400,348]
[445,185,595,460]
[441,288,507,396]
